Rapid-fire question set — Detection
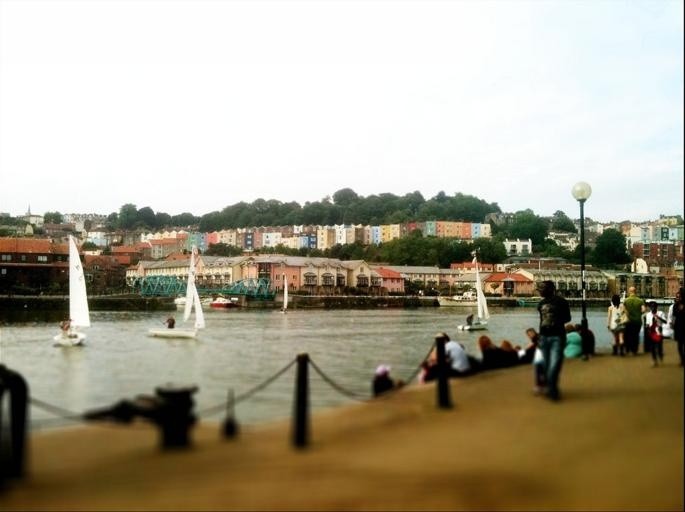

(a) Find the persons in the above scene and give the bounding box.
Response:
[161,314,175,327]
[367,321,598,400]
[607,282,684,369]
[533,279,572,404]
[60,318,72,339]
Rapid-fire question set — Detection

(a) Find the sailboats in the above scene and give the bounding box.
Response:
[456,251,492,331]
[52,233,94,350]
[173,294,236,311]
[149,245,209,341]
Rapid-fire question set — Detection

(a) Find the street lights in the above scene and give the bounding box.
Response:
[570,181,596,353]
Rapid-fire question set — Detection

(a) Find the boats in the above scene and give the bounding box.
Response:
[436,289,485,309]
[516,297,542,308]
